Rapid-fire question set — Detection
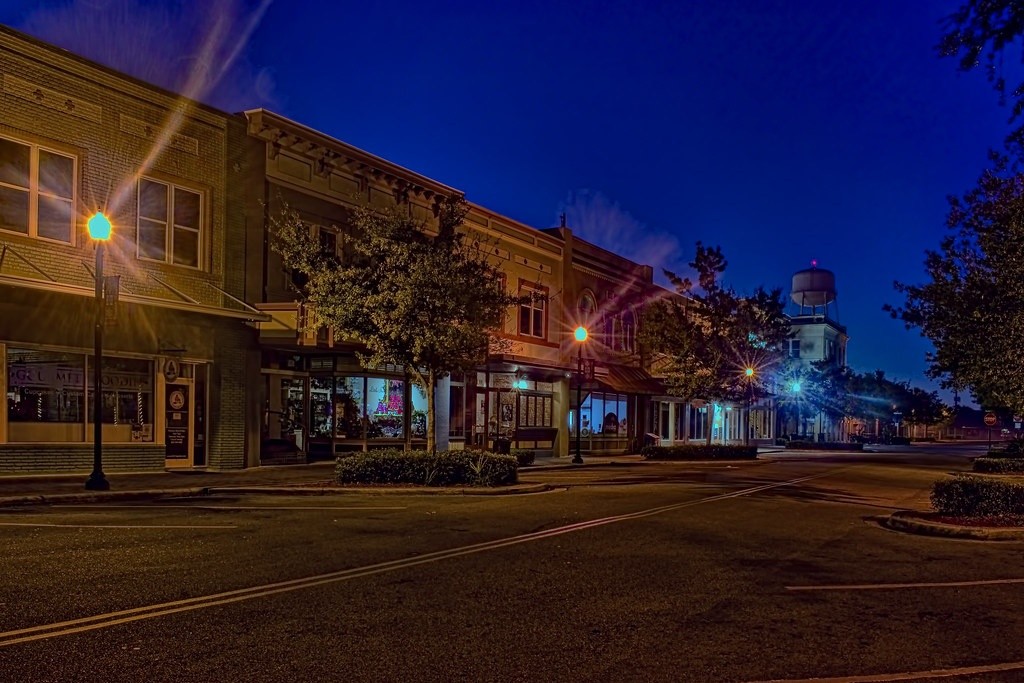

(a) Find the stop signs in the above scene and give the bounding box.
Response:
[984,413,997,425]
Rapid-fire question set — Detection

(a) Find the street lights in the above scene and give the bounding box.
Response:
[793,384,800,438]
[85,203,111,490]
[572,327,588,464]
[746,366,754,444]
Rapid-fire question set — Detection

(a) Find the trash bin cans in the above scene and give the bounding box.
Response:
[492,437,511,454]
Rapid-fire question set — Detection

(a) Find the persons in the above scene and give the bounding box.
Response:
[751,425,754,438]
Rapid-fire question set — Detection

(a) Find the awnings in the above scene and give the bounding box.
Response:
[586,363,666,396]
